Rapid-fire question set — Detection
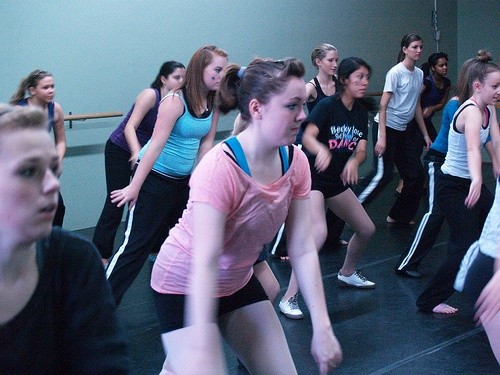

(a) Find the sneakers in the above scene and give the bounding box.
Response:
[337,272,376,289]
[279,298,303,319]
[403,270,423,277]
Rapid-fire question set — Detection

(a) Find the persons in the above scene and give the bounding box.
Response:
[151,56,342,375]
[230,112,280,366]
[104,45,230,305]
[0,104,129,375]
[10,70,66,228]
[269,44,349,259]
[395,49,500,375]
[93,61,187,269]
[279,56,382,320]
[393,52,451,198]
[355,34,432,225]
